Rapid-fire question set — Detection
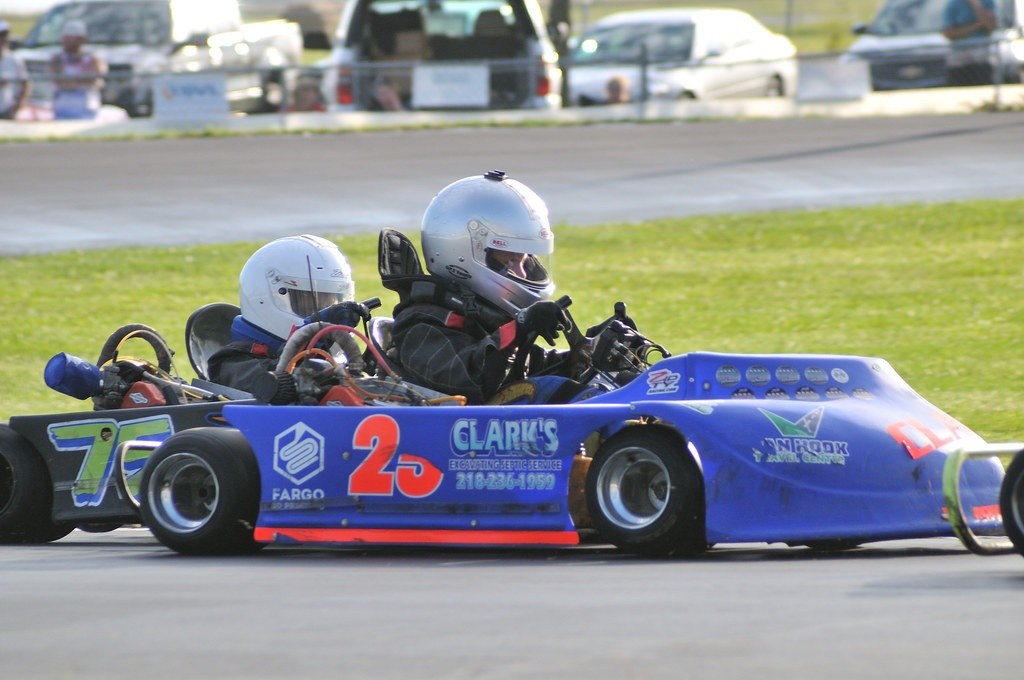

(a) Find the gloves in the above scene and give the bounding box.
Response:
[517,301,571,347]
[302,301,371,332]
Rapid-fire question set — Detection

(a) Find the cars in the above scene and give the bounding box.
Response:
[837,1,1024,93]
[566,9,801,107]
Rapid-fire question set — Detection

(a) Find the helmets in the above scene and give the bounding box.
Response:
[239,234,355,342]
[420,169,556,315]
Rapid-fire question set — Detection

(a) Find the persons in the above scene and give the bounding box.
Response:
[391,169,602,405]
[941,0,998,85]
[208,233,370,407]
[52,20,108,120]
[0,21,29,120]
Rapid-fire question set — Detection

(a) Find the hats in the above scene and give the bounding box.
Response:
[62,19,88,36]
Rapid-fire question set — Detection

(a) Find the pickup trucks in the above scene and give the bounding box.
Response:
[12,0,305,120]
[325,0,565,111]
